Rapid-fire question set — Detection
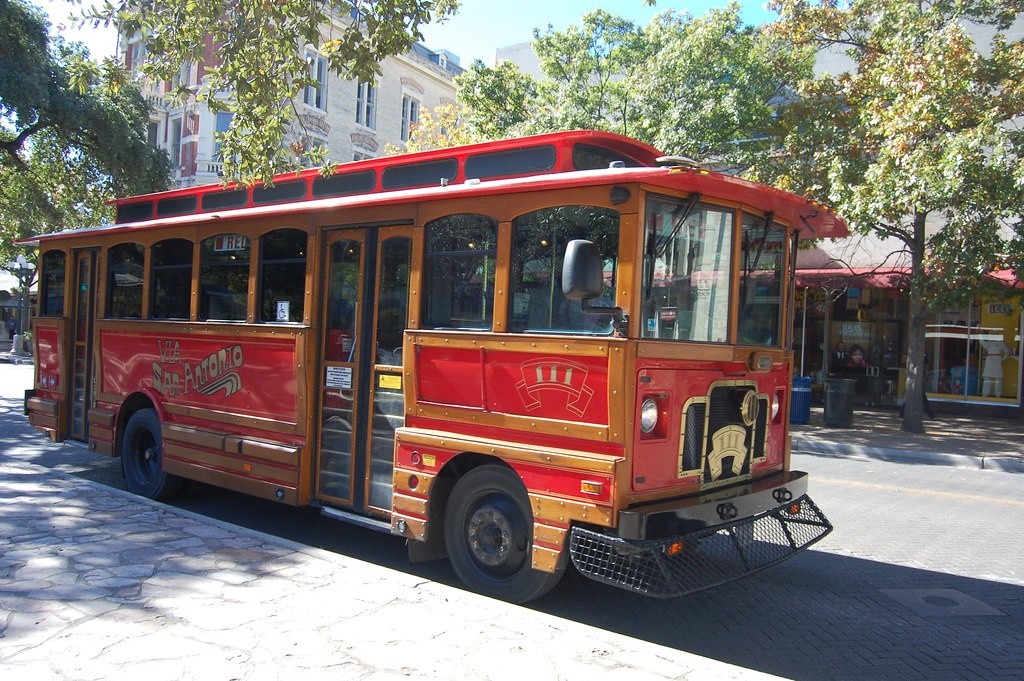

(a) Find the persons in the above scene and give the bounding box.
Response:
[832,340,867,367]
[977,325,1012,397]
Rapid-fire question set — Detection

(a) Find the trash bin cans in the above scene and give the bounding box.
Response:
[822,378,856,428]
[789,376,815,425]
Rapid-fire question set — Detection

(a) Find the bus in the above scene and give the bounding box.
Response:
[9,130,851,610]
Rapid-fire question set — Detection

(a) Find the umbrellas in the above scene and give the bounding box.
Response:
[0,299,33,308]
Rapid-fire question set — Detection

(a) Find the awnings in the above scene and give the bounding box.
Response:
[465,267,1024,295]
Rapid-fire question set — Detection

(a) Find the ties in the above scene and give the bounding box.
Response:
[840,353,842,360]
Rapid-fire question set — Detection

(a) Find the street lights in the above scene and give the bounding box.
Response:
[9,254,36,355]
